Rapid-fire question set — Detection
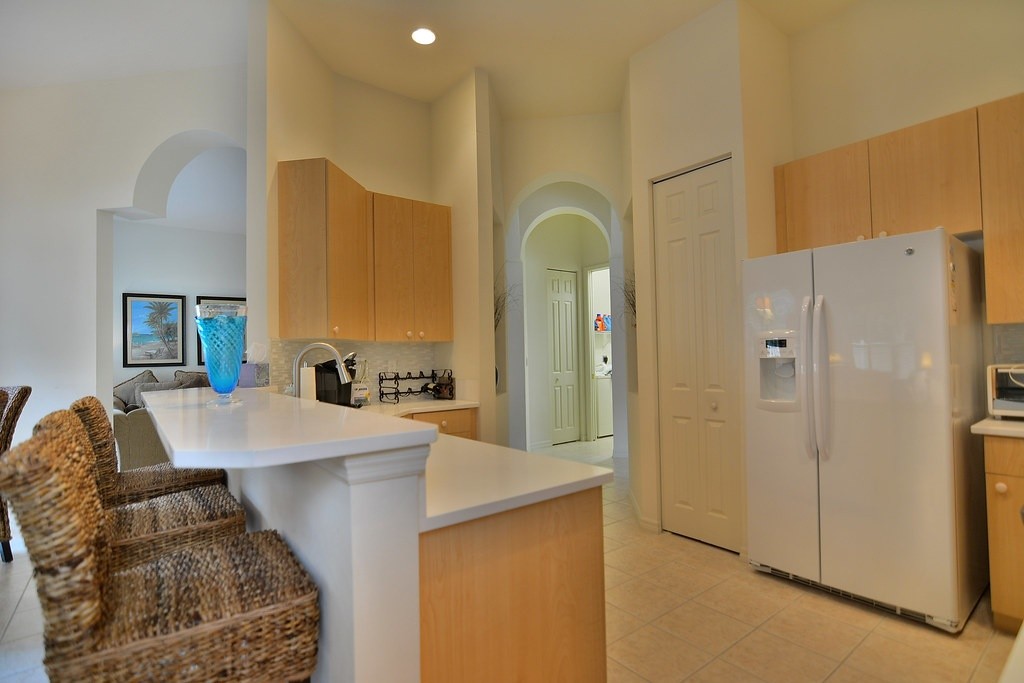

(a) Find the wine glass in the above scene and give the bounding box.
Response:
[194,304,248,409]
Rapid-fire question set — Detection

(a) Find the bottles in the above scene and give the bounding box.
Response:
[424,383,448,395]
[351,360,371,405]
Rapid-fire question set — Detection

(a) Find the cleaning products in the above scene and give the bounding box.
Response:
[603,314,611,332]
[595,314,604,332]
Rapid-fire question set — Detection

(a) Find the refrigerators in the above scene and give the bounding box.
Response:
[741,226,993,634]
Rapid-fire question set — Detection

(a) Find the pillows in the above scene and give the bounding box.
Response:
[112,369,157,404]
[174,370,210,388]
[134,380,183,406]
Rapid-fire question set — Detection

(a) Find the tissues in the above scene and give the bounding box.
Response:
[239,342,269,389]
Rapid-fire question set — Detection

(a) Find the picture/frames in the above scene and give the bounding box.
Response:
[122,293,186,367]
[196,295,249,366]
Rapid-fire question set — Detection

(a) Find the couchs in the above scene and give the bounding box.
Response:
[113,409,171,472]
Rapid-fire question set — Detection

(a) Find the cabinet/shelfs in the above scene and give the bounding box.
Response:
[278,157,369,341]
[984,434,1024,636]
[360,397,613,683]
[773,105,980,254]
[369,191,453,343]
[977,92,1024,324]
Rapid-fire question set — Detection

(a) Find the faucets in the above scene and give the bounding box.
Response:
[292,343,352,399]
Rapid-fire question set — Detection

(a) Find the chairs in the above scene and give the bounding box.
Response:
[0,429,320,683]
[70,395,228,509]
[33,410,246,572]
[0,385,32,562]
